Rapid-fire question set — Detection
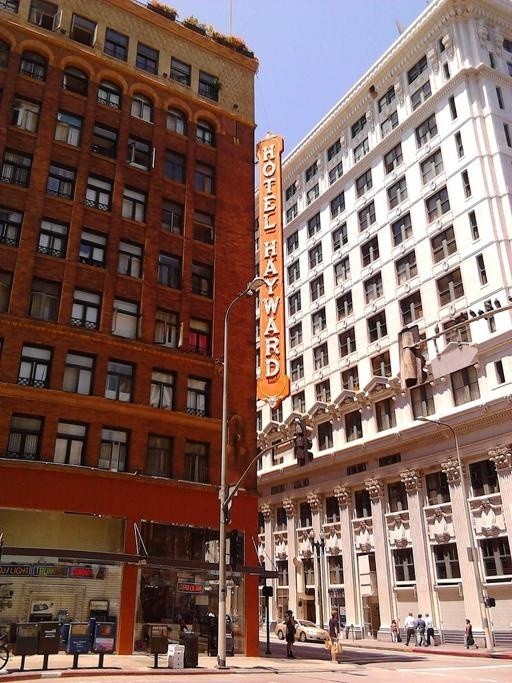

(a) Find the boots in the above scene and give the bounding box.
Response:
[287,650,295,657]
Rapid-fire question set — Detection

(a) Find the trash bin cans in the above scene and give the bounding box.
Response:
[179,632,198,668]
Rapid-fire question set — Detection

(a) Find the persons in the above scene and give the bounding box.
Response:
[414,613,430,647]
[403,612,419,647]
[325,609,340,664]
[462,618,479,650]
[286,616,297,657]
[285,609,294,654]
[390,621,398,646]
[389,618,396,642]
[424,612,438,646]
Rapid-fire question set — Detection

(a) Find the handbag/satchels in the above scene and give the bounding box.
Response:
[468,636,474,645]
[287,622,296,633]
[334,642,342,654]
[325,640,331,650]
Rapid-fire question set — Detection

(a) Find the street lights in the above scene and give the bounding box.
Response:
[416,415,494,651]
[308,528,326,629]
[217,277,299,671]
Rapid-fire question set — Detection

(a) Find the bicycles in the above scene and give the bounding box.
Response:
[0,632,10,671]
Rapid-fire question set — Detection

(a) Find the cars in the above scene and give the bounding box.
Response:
[274,619,329,642]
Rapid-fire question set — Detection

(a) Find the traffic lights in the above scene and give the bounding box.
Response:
[304,418,315,466]
[418,325,427,387]
